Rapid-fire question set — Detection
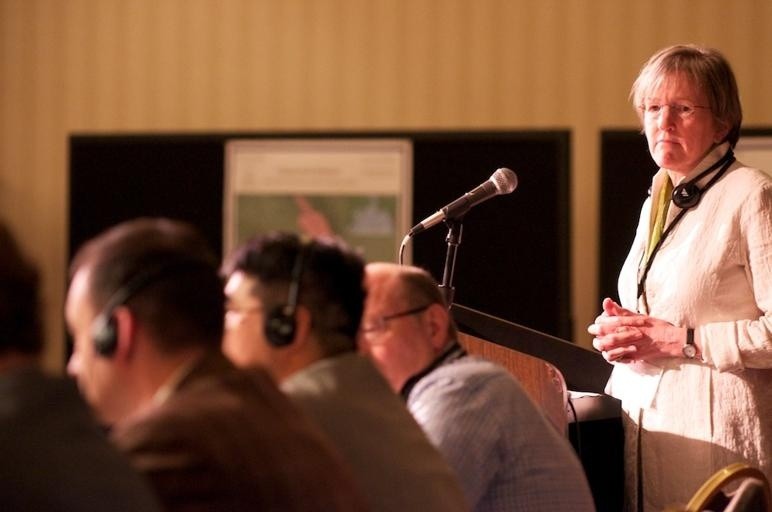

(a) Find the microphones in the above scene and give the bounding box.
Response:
[410,166,517,234]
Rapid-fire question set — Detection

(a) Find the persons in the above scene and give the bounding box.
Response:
[358,261,597,511]
[59,215,374,510]
[0,219,167,510]
[588,42,771,511]
[220,228,475,510]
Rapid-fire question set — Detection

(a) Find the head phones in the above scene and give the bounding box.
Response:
[265,247,311,346]
[674,152,738,209]
[92,258,167,357]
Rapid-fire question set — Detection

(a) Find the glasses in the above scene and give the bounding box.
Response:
[643,101,712,117]
[361,305,430,339]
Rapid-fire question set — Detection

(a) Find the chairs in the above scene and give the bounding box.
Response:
[686,460,770,512]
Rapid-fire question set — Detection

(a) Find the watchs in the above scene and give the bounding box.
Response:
[682,327,697,359]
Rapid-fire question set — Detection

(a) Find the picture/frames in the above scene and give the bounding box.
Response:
[221,140,414,268]
